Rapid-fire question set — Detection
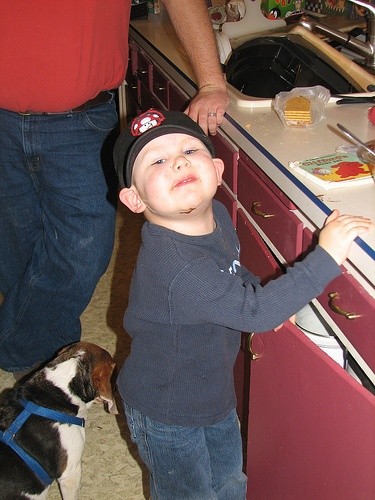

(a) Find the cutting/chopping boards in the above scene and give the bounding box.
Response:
[229,25,375,94]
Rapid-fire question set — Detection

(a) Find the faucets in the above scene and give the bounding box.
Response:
[298,0,375,67]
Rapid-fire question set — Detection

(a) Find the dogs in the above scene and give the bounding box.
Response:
[0,341,122,500]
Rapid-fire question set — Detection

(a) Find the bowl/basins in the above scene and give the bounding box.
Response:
[357,140,375,183]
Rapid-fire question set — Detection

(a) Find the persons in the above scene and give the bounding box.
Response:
[114,108,372,500]
[0,1,231,382]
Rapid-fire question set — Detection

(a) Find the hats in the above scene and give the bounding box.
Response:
[113,109,215,189]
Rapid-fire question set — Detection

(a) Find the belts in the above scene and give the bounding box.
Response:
[18,90,112,116]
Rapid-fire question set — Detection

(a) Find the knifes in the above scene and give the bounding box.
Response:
[336,96,375,104]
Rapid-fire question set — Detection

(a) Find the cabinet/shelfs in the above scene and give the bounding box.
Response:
[125,37,375,500]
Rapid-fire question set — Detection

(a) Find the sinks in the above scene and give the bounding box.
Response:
[222,34,359,107]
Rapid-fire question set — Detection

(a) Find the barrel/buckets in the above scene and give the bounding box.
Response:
[294,299,366,385]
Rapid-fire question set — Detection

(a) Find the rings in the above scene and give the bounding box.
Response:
[208,113,216,116]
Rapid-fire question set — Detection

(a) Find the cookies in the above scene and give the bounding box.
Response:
[284,98,312,125]
